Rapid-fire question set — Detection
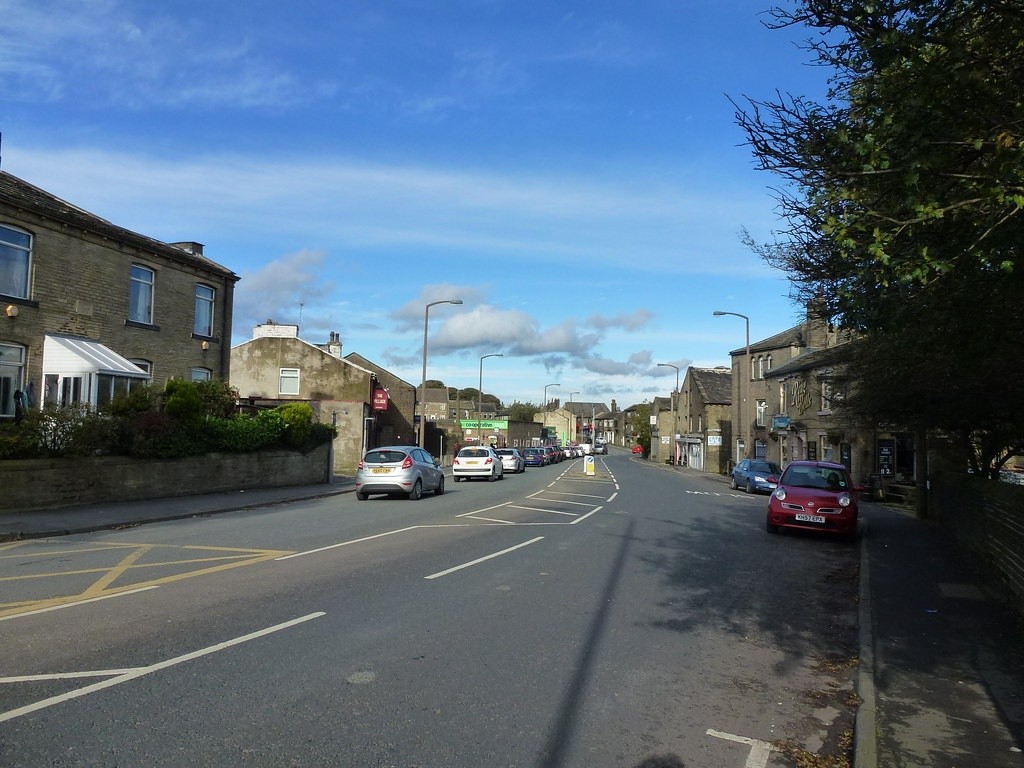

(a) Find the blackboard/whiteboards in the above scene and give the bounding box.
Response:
[878,446,894,477]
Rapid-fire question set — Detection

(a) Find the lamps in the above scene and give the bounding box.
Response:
[786,424,799,434]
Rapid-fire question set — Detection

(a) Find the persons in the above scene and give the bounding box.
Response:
[828,472,839,481]
[454,441,459,458]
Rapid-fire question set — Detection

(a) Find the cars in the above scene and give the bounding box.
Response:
[354,444,445,501]
[632,444,642,455]
[767,459,863,540]
[492,447,525,474]
[730,458,784,495]
[452,445,503,482]
[521,435,608,467]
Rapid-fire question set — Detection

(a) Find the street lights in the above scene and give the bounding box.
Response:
[713,310,750,459]
[418,297,466,447]
[657,363,679,467]
[477,354,505,446]
[570,390,580,445]
[543,383,560,427]
